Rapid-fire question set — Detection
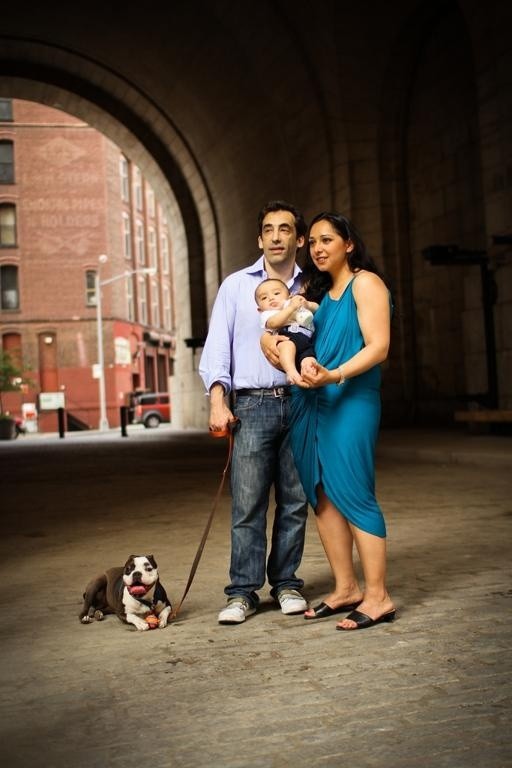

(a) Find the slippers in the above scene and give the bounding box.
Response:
[336,609,395,631]
[304,602,361,620]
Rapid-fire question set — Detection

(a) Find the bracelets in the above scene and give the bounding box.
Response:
[337,365,345,387]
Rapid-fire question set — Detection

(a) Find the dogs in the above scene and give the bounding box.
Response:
[77,552,174,633]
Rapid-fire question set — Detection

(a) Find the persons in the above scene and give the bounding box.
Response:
[252,277,321,387]
[195,200,307,625]
[258,210,398,631]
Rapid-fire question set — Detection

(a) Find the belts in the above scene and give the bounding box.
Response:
[232,385,294,397]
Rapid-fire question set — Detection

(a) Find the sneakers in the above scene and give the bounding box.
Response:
[218,600,258,625]
[274,589,309,615]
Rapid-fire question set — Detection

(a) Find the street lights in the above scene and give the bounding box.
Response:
[94,254,156,430]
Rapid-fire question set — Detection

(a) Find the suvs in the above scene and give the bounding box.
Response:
[134,393,170,427]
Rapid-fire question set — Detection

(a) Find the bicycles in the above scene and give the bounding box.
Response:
[0,399,18,439]
